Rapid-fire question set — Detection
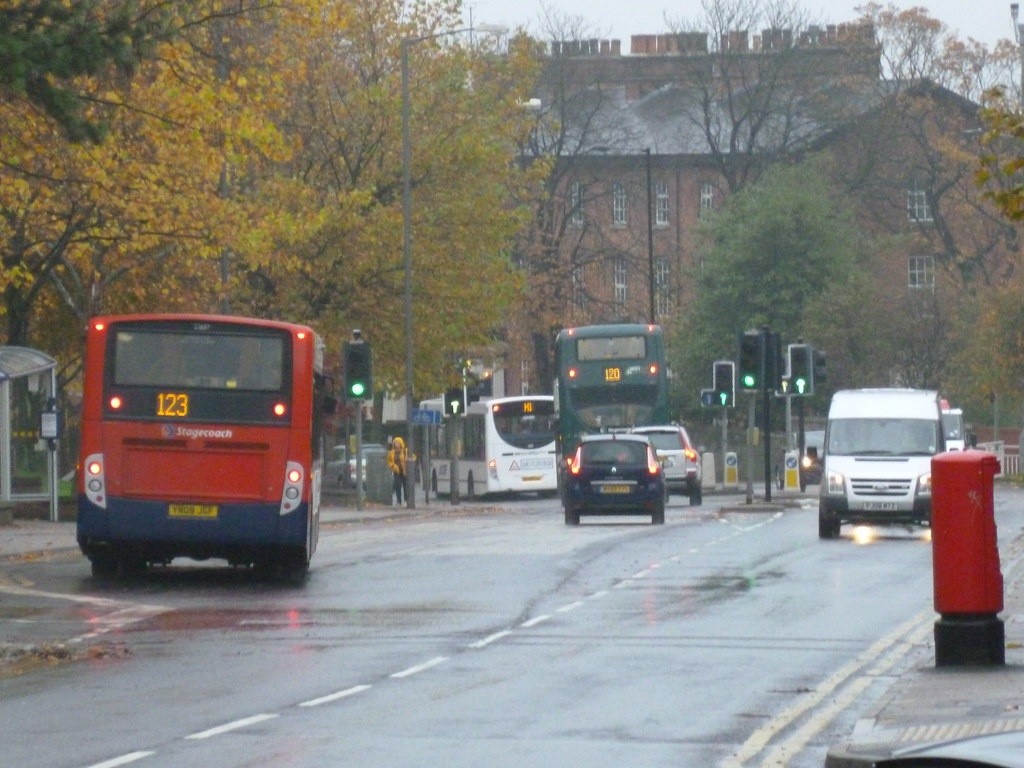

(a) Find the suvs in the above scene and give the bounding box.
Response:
[623,420,706,505]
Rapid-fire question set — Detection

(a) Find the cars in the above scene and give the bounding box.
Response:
[559,433,673,526]
[777,445,826,492]
[325,443,390,489]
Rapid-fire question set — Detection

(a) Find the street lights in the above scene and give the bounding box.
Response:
[402,21,511,511]
[593,143,656,326]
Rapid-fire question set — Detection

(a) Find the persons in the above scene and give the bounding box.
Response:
[389,437,416,503]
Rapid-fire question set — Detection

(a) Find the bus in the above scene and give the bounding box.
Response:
[413,394,557,502]
[76,312,338,577]
[550,324,679,457]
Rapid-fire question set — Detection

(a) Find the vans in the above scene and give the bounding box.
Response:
[815,387,961,539]
[942,407,978,453]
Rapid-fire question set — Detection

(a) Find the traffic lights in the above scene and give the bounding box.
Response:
[811,346,829,388]
[344,340,373,402]
[788,344,814,398]
[738,329,765,392]
[442,367,468,417]
[713,361,735,408]
[701,389,714,408]
[776,374,788,397]
